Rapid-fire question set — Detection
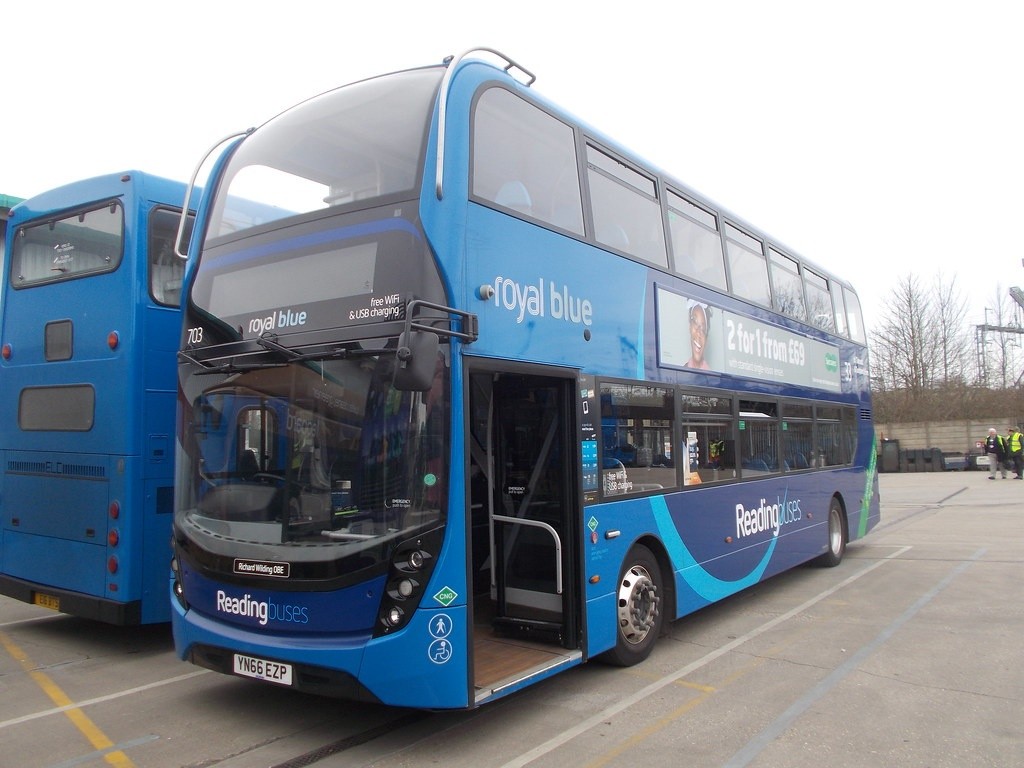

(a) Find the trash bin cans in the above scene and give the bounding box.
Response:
[882,439,898,473]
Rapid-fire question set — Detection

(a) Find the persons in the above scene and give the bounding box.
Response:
[984,428,1024,480]
[709,436,724,470]
[682,299,714,372]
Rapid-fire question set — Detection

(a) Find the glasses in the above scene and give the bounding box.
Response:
[691,316,707,337]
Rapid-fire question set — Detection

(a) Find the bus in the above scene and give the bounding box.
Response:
[1,170,302,627]
[169,48,880,711]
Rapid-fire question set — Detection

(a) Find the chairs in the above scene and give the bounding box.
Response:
[322,168,380,208]
[604,446,808,493]
[494,178,530,216]
[607,222,629,252]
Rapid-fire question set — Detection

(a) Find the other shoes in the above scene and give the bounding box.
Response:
[988,475,995,480]
[1013,476,1022,479]
[1002,477,1006,479]
